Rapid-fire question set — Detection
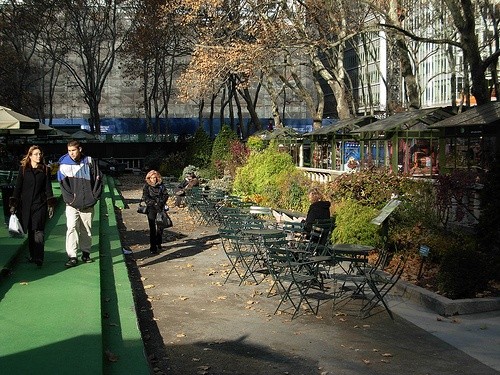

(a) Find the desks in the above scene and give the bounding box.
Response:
[327,244,374,298]
[240,229,283,261]
[276,256,331,293]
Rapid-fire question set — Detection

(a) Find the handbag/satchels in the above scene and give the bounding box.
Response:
[161,212,172,228]
[8,214,24,238]
[137,204,147,215]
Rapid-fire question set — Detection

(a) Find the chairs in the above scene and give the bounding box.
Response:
[0,170,19,192]
[190,186,407,320]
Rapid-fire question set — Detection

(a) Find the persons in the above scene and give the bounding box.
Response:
[140,170,169,255]
[10,145,55,266]
[174,172,198,207]
[57,140,104,266]
[298,190,331,240]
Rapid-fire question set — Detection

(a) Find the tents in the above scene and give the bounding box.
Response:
[245,100,500,181]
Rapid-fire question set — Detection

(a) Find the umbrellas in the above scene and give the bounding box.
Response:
[1,107,95,157]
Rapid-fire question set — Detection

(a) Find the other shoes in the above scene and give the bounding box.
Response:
[65,259,77,266]
[81,255,92,263]
[158,242,162,249]
[36,260,41,265]
[150,249,155,254]
[30,257,35,263]
